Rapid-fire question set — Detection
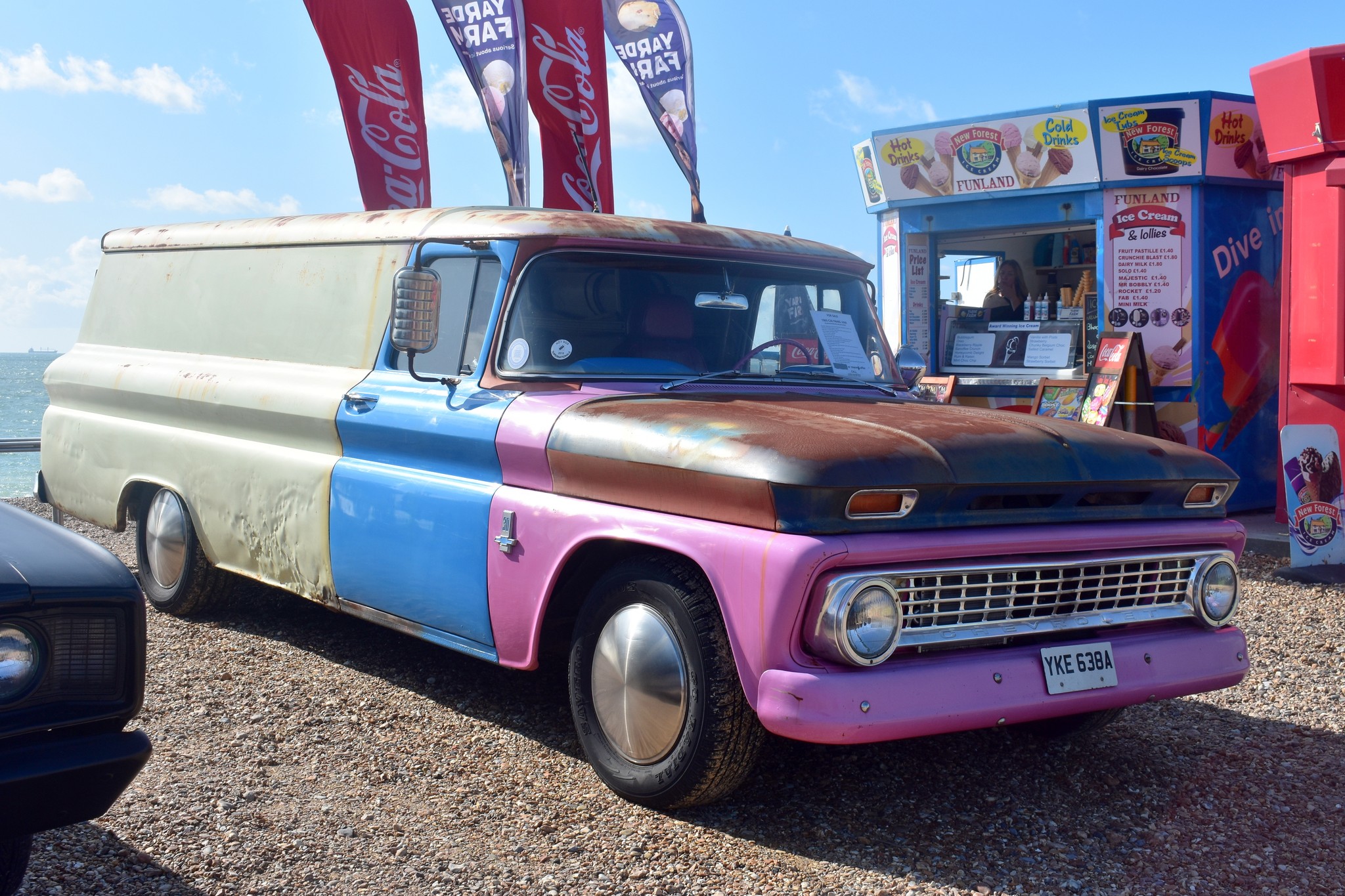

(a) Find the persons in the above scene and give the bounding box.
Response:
[982,259,1033,323]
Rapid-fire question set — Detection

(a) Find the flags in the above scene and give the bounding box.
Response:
[304,0,701,218]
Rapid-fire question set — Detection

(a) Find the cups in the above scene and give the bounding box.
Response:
[1046,271,1058,314]
[1063,283,1071,288]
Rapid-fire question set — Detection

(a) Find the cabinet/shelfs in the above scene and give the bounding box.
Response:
[939,318,1083,378]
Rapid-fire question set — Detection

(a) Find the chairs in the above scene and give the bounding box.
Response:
[627,304,709,375]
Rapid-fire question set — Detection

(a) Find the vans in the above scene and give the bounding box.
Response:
[34,204,1248,808]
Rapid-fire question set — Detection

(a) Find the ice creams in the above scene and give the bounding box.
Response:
[1284,447,1341,509]
[900,123,1074,197]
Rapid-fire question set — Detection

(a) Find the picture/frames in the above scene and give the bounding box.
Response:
[1092,331,1134,368]
[1076,368,1123,426]
[916,375,957,404]
[1030,377,1087,422]
